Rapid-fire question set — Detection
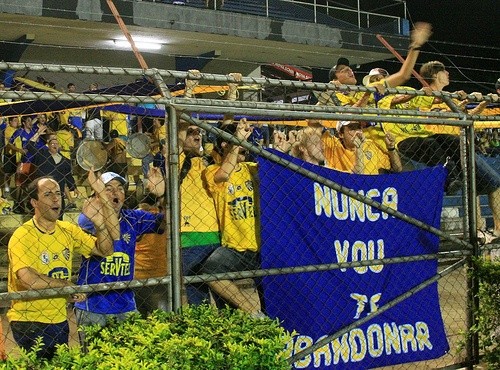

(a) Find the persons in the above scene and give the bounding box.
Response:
[198,118,288,322]
[417,60,500,238]
[273,127,366,176]
[494,77,500,96]
[162,65,243,317]
[130,190,168,320]
[466,128,500,158]
[7,174,116,370]
[1,65,225,212]
[311,22,431,114]
[358,67,477,198]
[71,166,166,357]
[304,80,405,175]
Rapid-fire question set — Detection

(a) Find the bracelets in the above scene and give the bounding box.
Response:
[387,147,396,151]
[408,44,422,52]
[95,224,107,232]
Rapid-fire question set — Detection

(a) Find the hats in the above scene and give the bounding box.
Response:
[362,68,388,84]
[100,172,127,185]
[336,112,360,130]
[329,57,350,79]
